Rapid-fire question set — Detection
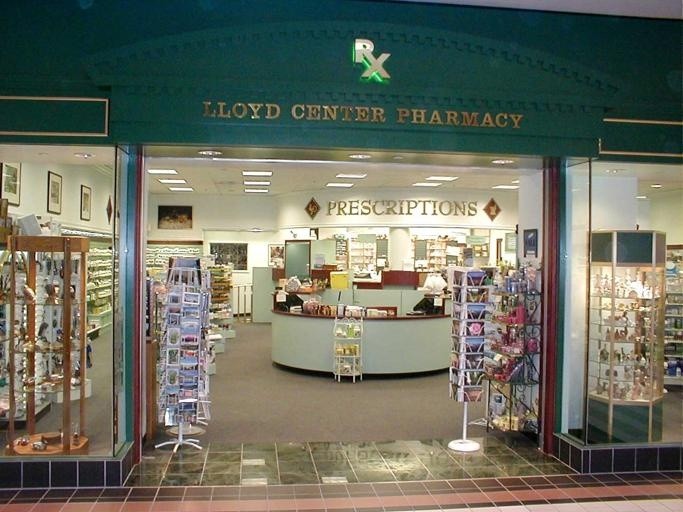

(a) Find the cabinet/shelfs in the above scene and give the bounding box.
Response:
[587,229,683,445]
[0,198,119,455]
[146,240,235,374]
[485,257,542,453]
[252,233,491,278]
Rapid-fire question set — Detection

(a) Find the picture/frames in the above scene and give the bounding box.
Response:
[48,171,62,215]
[158,205,193,229]
[209,242,248,273]
[0,161,22,207]
[523,228,538,258]
[80,184,92,221]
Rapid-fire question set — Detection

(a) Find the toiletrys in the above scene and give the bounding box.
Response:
[592,268,663,402]
